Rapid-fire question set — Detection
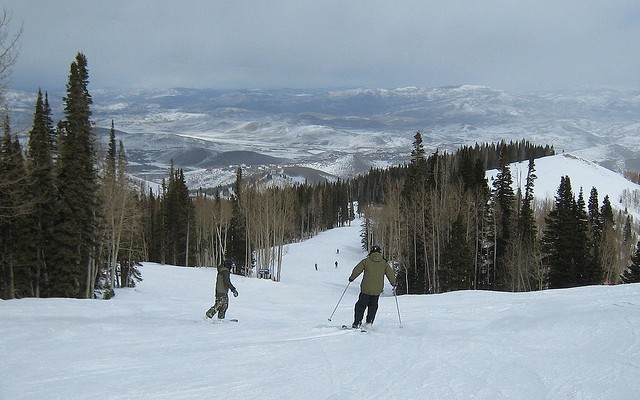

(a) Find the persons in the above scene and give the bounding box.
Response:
[315,263,318,270]
[206,260,239,320]
[349,246,397,328]
[337,248,339,253]
[335,261,338,267]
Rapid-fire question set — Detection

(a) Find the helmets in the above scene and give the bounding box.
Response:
[371,245,382,252]
[224,260,233,267]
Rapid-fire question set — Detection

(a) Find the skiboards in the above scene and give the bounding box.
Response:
[193,319,239,323]
[340,324,371,332]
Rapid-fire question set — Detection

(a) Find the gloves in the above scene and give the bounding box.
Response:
[232,288,238,297]
[349,277,353,282]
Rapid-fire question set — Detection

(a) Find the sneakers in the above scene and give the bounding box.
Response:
[352,323,360,328]
[204,316,214,323]
